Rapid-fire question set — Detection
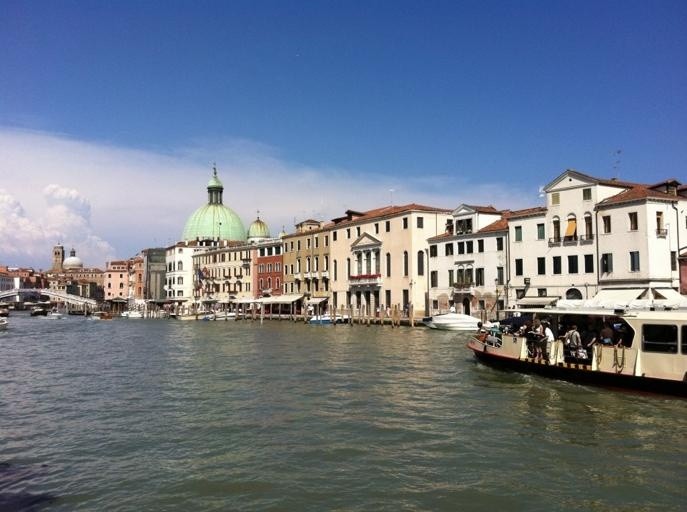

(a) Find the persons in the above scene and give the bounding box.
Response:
[474,321,488,343]
[586,327,600,349]
[599,324,615,347]
[557,325,583,358]
[528,318,545,359]
[540,322,555,359]
[611,326,626,343]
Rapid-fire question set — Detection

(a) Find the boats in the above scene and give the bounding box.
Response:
[0,308,9,330]
[415,305,687,398]
[122,309,243,322]
[310,314,348,323]
[29,308,112,320]
